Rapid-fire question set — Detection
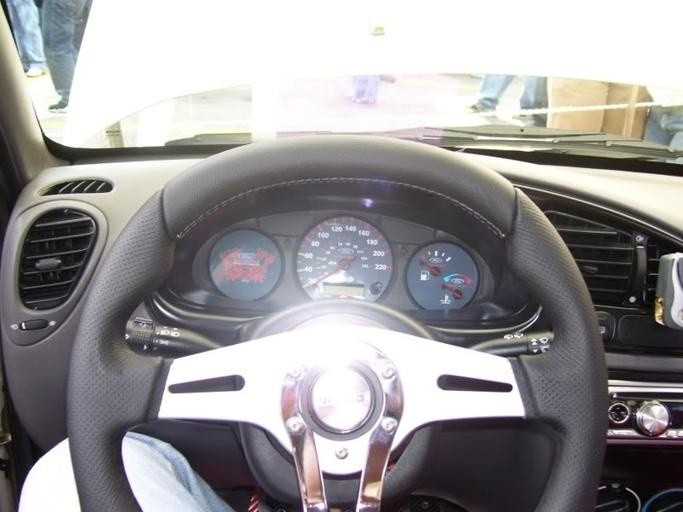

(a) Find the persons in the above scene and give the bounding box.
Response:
[638,93,682,152]
[466,72,537,121]
[33,0,93,114]
[4,0,47,78]
[542,76,653,141]
[11,430,243,512]
